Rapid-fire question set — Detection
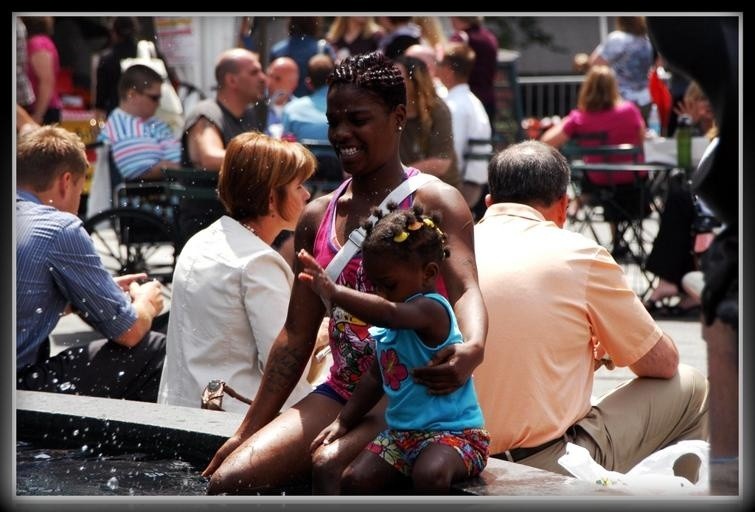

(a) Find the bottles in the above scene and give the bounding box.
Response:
[676,116,692,171]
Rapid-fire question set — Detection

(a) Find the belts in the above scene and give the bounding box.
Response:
[490,426,582,465]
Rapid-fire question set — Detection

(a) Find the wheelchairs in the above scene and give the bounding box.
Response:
[79,142,220,329]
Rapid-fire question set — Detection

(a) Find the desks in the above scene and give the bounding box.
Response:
[557,127,681,311]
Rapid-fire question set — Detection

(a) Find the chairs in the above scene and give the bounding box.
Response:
[161,164,223,278]
[456,136,500,216]
[301,139,346,200]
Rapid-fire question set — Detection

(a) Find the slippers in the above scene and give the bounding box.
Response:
[645,299,704,320]
